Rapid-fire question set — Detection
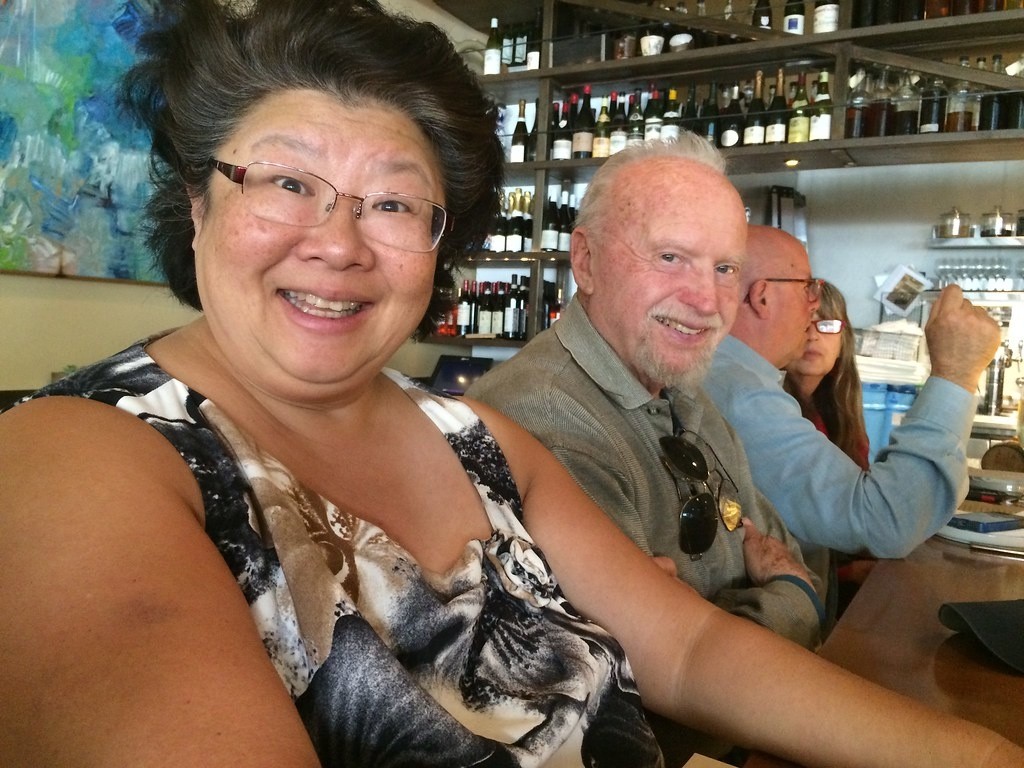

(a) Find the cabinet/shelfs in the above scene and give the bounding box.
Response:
[401,0,1023,399]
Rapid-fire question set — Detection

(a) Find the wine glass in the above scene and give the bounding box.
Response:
[936,256,1024,292]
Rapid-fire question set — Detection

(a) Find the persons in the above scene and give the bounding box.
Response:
[465,136,826,652]
[785,283,875,591]
[702,226,1001,560]
[0,0,1024,768]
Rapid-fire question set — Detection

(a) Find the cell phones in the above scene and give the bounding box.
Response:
[945,510,1024,534]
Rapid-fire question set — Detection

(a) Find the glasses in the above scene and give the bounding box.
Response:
[743,278,824,304]
[208,158,457,252]
[677,426,744,531]
[806,318,846,334]
[659,435,718,562]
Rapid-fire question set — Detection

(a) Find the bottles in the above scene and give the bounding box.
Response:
[434,276,564,339]
[550,0,1024,162]
[481,188,578,251]
[481,4,543,162]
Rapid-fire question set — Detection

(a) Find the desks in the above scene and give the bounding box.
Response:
[744,467,1024,767]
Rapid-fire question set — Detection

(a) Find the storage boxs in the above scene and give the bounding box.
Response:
[860,382,924,467]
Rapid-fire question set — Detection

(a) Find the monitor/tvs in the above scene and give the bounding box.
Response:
[427,354,495,397]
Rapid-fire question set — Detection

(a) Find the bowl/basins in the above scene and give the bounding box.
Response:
[980,203,1014,237]
[937,205,971,238]
